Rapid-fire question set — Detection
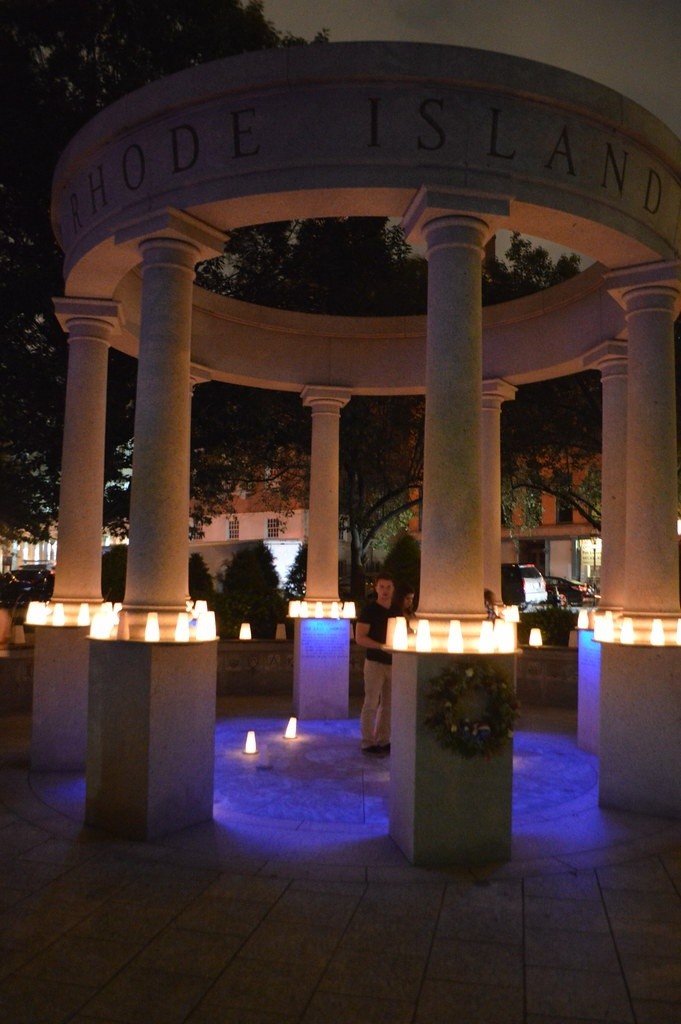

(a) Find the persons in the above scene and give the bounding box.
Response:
[397,582,415,614]
[355,574,405,757]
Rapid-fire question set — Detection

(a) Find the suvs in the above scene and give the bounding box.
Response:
[502,563,548,605]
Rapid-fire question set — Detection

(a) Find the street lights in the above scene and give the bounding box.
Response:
[593,542,597,585]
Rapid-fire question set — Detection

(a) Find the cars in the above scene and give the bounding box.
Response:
[543,576,592,606]
[38,566,56,582]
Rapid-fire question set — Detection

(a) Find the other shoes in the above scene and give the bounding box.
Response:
[362,745,385,755]
[381,743,390,753]
